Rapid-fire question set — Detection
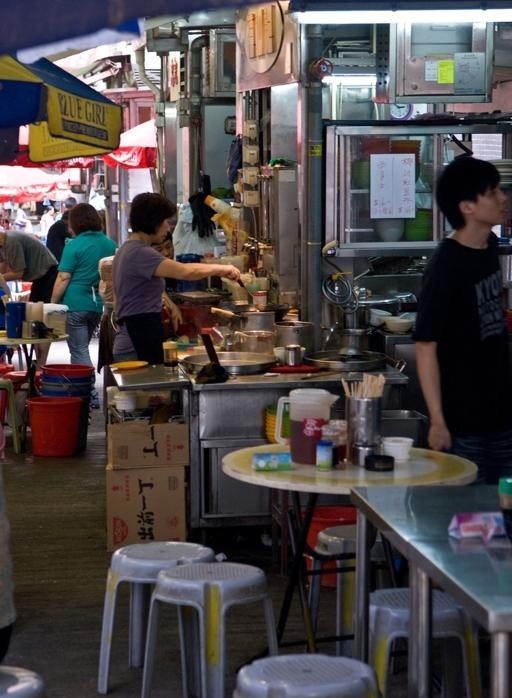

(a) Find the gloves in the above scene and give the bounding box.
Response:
[188,192,216,238]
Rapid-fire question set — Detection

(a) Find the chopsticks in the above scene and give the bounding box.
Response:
[342,368,387,399]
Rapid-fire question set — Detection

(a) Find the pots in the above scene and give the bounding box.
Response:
[211,308,316,352]
[335,325,372,350]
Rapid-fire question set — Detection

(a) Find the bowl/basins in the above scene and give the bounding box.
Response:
[377,208,433,242]
[274,347,305,365]
[366,308,390,325]
[265,403,289,444]
[351,135,422,189]
[385,317,413,333]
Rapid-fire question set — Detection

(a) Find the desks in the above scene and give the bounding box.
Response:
[0,331,70,441]
[350,484,512,698]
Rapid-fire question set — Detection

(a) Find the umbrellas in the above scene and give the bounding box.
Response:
[59,167,90,185]
[0,125,94,168]
[99,118,160,169]
[0,57,123,164]
[0,165,67,203]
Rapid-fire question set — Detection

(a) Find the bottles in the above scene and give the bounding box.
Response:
[203,194,242,219]
[315,419,347,469]
[163,342,180,373]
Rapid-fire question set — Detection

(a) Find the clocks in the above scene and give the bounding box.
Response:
[391,104,413,120]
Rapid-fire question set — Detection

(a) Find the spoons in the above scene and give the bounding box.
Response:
[338,346,363,362]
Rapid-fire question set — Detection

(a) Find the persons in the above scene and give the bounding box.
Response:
[411,156,512,484]
[1,190,241,410]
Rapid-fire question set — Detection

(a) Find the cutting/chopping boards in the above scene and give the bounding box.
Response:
[179,291,220,299]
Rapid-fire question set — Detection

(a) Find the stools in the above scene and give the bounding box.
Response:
[1,372,28,459]
[233,654,379,698]
[0,666,44,698]
[0,378,21,454]
[369,588,482,698]
[141,562,279,698]
[98,541,215,694]
[307,526,398,656]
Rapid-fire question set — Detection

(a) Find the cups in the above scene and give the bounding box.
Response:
[253,290,268,310]
[22,320,38,340]
[344,392,383,450]
[383,437,415,463]
[5,301,22,338]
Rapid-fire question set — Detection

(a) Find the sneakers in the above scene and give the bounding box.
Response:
[90,393,99,409]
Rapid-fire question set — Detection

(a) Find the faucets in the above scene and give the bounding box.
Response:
[332,273,354,297]
[242,237,273,256]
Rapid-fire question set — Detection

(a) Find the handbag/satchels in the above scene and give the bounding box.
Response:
[14,218,27,227]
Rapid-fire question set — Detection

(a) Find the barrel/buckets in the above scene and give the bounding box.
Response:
[301,504,357,588]
[0,364,97,461]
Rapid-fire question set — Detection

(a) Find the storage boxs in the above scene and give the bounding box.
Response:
[106,424,190,470]
[106,466,186,552]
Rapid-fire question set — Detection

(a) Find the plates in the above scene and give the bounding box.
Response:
[109,360,148,371]
[485,158,512,190]
